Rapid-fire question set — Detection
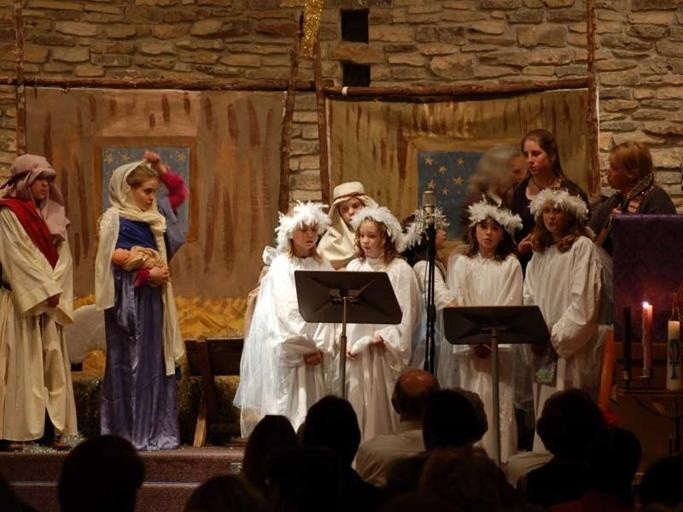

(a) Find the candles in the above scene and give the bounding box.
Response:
[667,321,682,391]
[622,305,633,376]
[641,304,652,376]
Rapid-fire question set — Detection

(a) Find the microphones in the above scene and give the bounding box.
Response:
[422,191,437,232]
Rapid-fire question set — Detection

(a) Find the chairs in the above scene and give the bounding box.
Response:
[182,340,201,448]
[62,294,109,371]
[196,337,243,449]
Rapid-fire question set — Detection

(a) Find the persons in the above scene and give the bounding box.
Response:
[514,387,683,512]
[0,154,83,451]
[57,432,147,512]
[232,129,677,469]
[185,395,391,512]
[111,247,170,284]
[90,149,193,453]
[356,370,516,512]
